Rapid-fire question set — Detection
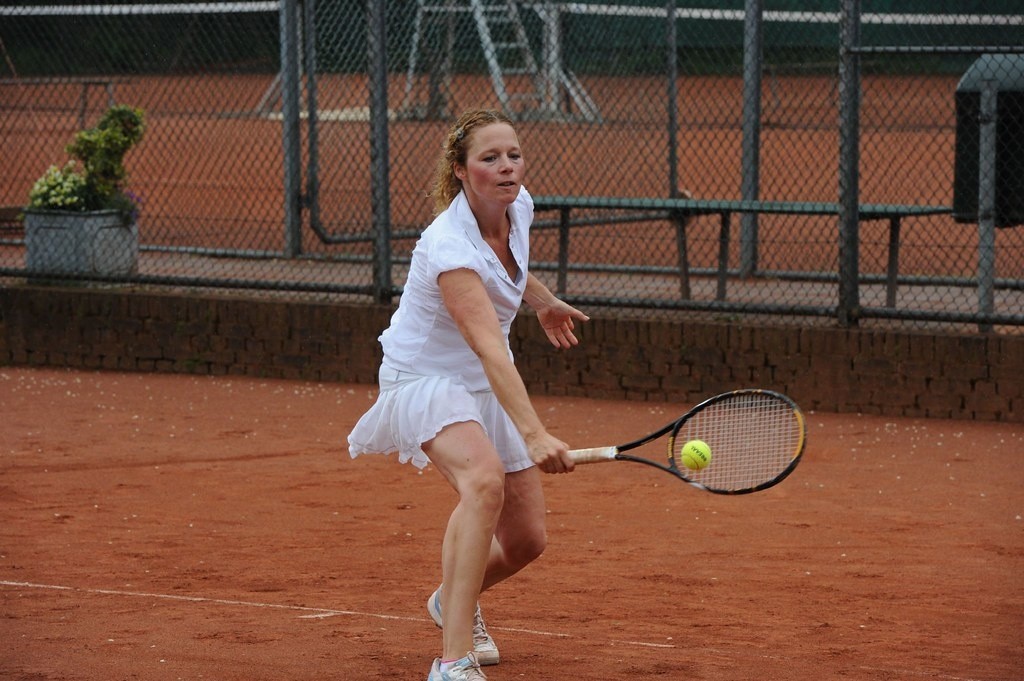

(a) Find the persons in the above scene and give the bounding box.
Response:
[346,105,589,678]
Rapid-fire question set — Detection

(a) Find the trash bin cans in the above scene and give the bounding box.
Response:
[948,53,1024,229]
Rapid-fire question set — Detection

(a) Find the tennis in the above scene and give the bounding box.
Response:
[680,439,711,471]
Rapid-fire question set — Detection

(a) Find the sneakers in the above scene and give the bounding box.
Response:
[426,582,500,666]
[427,651,488,681]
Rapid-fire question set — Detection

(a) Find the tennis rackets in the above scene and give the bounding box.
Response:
[566,388,809,496]
